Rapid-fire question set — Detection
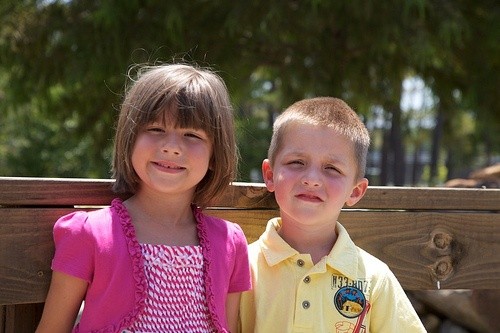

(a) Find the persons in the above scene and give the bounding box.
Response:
[36,59,254,333]
[237,97,427,333]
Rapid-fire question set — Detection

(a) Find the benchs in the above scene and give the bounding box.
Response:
[0,177,500,333]
